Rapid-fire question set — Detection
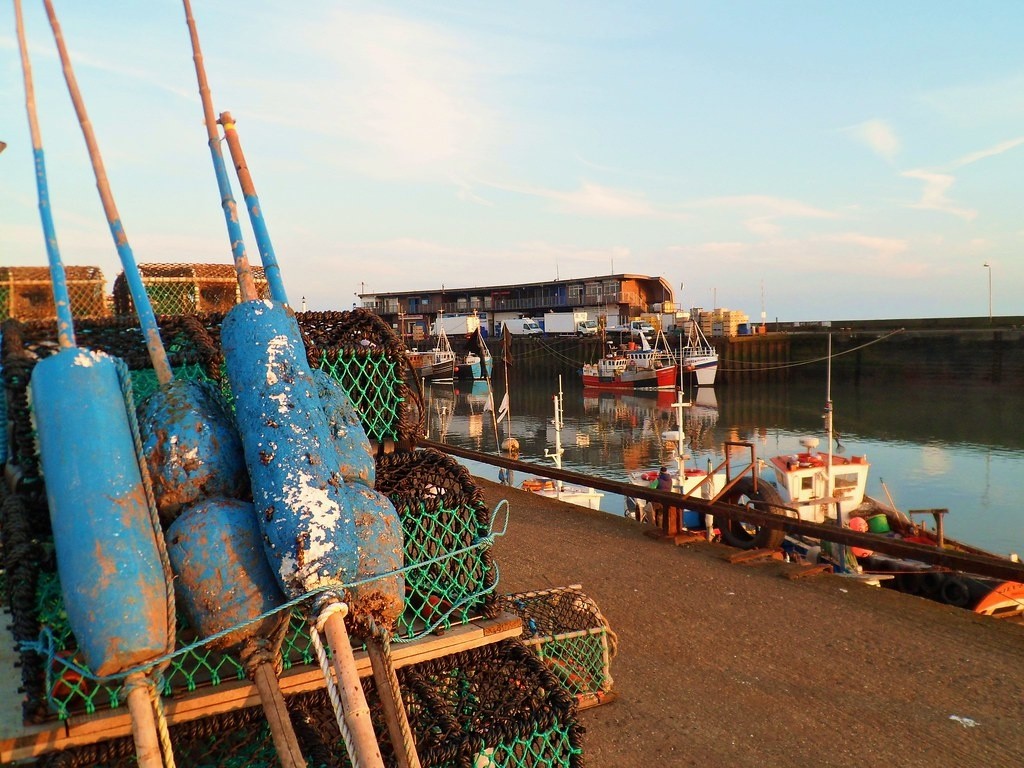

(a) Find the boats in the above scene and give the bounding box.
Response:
[582,319,677,389]
[624,333,895,587]
[425,308,457,382]
[455,309,493,380]
[607,305,719,388]
[522,374,605,511]
[770,332,1024,624]
[400,305,433,379]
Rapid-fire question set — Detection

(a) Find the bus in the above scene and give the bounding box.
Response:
[440,312,489,338]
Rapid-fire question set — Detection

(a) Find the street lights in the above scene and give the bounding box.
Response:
[984,264,992,323]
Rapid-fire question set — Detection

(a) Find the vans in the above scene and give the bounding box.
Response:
[501,318,543,338]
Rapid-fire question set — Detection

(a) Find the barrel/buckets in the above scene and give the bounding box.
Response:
[867,514,890,533]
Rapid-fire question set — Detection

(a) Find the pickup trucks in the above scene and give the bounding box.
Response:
[605,320,655,337]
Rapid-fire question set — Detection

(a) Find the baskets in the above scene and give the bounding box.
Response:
[4,310,425,470]
[114,264,275,317]
[505,583,617,714]
[0,266,109,319]
[29,451,502,721]
[28,640,584,768]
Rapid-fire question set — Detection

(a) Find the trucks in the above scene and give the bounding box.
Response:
[544,312,598,337]
[430,316,480,338]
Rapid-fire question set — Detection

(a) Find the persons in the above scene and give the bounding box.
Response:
[650,467,672,492]
